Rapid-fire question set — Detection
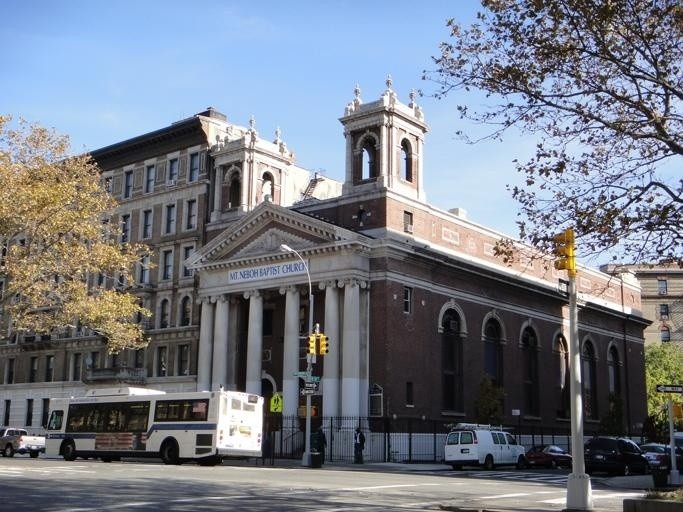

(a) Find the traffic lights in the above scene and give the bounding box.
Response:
[317,336,331,355]
[305,335,318,356]
[550,228,576,276]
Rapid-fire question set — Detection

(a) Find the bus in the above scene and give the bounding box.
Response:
[45,383,266,466]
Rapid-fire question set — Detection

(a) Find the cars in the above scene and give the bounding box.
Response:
[526,429,682,479]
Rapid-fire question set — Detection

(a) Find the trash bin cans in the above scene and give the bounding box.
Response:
[651,467,668,488]
[311,453,322,468]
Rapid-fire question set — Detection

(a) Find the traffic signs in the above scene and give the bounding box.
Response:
[655,383,682,394]
[292,371,320,395]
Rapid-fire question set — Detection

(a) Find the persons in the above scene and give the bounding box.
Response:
[354,427,365,464]
[317,428,327,464]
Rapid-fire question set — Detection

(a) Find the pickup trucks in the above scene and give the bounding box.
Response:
[0,427,47,458]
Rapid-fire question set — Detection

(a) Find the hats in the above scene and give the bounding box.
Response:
[356,428,360,431]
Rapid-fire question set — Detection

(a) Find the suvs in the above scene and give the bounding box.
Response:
[441,421,527,473]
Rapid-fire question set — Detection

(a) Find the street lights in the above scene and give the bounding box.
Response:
[278,240,318,464]
